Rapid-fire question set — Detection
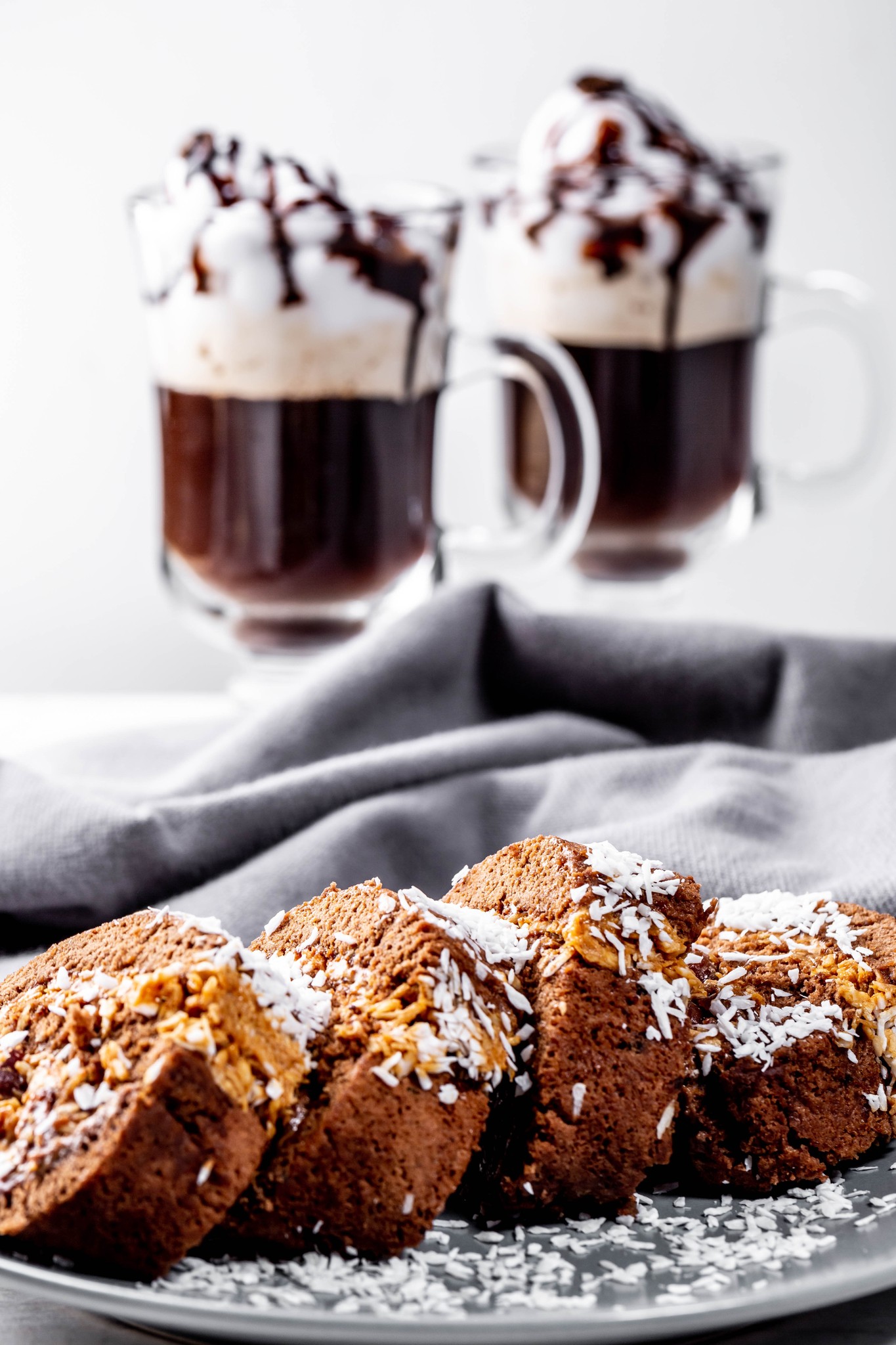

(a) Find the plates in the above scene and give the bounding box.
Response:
[0,1133,895,1343]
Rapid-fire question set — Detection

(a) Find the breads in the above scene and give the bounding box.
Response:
[0,836,896,1279]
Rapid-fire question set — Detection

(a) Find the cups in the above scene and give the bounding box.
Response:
[467,160,896,586]
[130,188,602,655]
